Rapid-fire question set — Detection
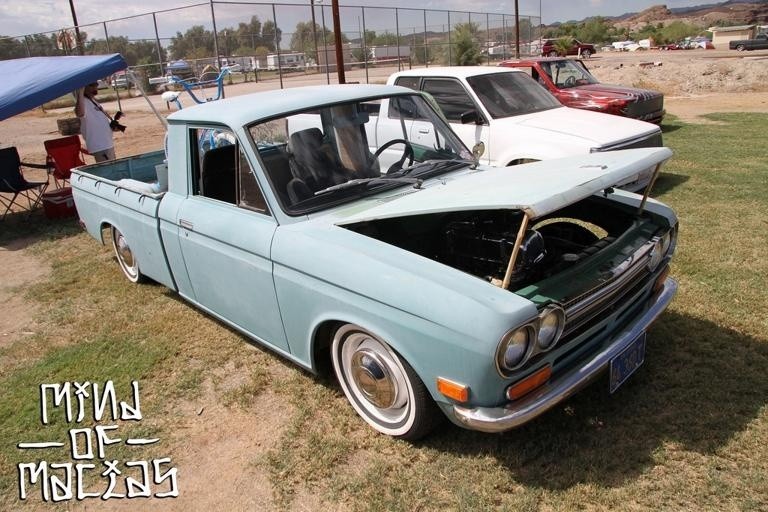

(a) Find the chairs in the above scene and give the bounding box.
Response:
[288,128,355,191]
[332,115,380,179]
[202,144,240,204]
[44,134,94,188]
[0,146,49,224]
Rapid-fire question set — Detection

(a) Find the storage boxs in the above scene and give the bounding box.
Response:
[42,187,78,219]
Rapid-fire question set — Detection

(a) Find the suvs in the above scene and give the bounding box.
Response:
[536,37,596,61]
[111,71,143,91]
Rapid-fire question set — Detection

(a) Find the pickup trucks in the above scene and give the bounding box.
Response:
[729,32,768,52]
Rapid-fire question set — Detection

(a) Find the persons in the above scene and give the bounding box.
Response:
[71,82,123,165]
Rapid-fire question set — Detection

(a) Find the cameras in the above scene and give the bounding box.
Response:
[109,111,127,133]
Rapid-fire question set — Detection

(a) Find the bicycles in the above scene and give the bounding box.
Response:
[146,64,246,165]
